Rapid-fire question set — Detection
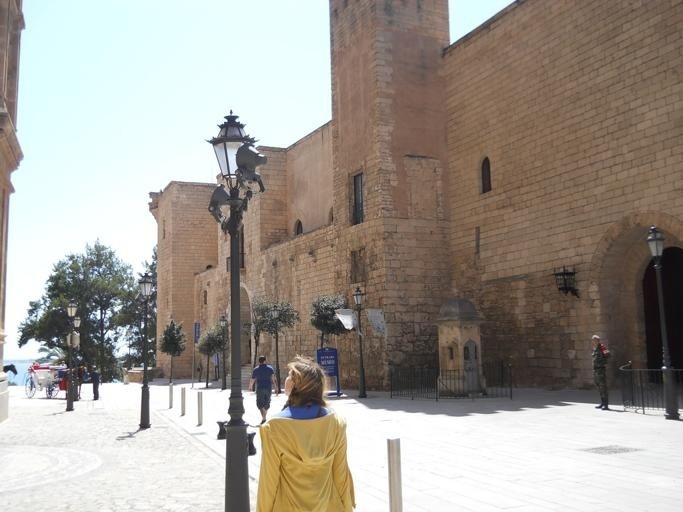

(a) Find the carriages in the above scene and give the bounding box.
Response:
[24,362,85,399]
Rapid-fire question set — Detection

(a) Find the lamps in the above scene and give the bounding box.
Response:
[554,265,580,299]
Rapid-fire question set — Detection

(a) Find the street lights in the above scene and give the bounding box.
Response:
[270,305,281,393]
[138,273,152,428]
[66,298,82,411]
[352,286,367,398]
[646,224,679,420]
[219,316,228,390]
[205,110,264,512]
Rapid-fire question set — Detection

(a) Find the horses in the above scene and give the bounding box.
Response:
[3,364,17,375]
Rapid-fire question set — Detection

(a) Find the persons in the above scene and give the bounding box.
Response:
[197,358,203,382]
[256,353,356,512]
[89,365,100,400]
[60,360,67,369]
[591,334,611,409]
[248,355,279,424]
